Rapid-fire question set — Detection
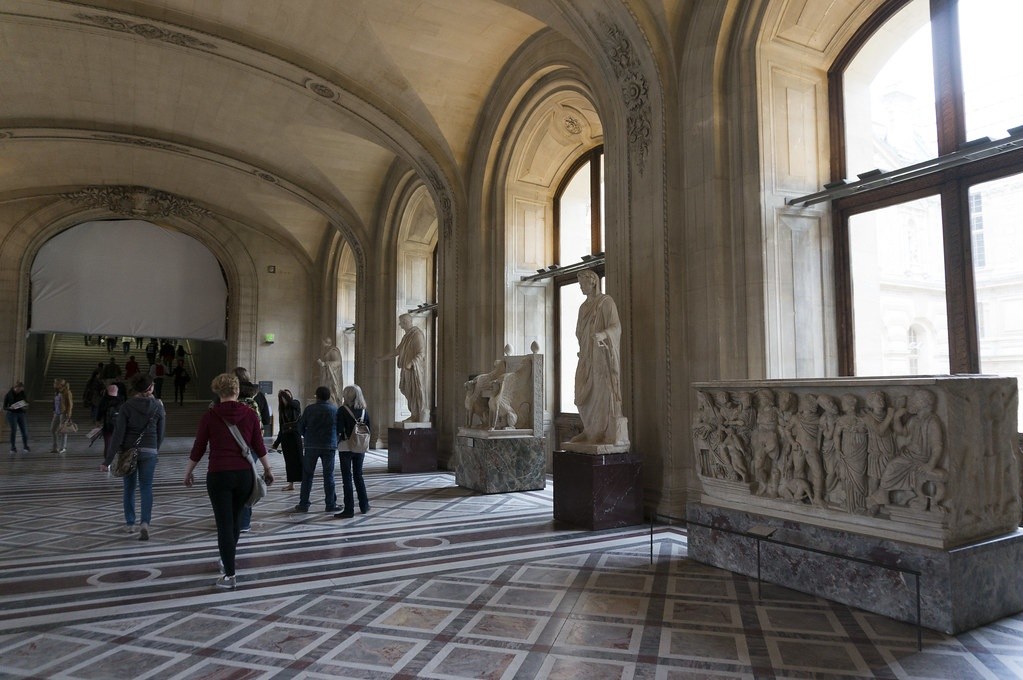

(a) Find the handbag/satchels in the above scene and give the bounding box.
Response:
[56,417,78,434]
[84,387,92,407]
[250,474,267,507]
[109,446,138,478]
[181,371,192,383]
[281,410,302,436]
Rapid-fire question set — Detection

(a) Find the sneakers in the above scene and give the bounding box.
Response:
[137,523,150,541]
[217,558,226,574]
[216,576,236,588]
[121,524,136,533]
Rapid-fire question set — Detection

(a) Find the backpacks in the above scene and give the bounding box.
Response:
[102,399,122,432]
[343,406,371,454]
[155,364,164,376]
[127,362,136,375]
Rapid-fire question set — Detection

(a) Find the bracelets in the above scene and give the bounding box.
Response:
[263,467,271,471]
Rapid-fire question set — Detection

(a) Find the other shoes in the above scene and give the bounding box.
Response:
[334,511,355,518]
[51,449,66,454]
[362,505,371,514]
[295,505,309,512]
[240,525,251,533]
[282,486,294,491]
[23,447,30,453]
[10,449,17,454]
[325,505,343,512]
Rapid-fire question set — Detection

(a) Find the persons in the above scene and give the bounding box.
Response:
[690,389,949,518]
[570,270,629,446]
[183,374,275,589]
[82,333,193,418]
[3,382,31,454]
[378,313,430,423]
[209,366,304,492]
[287,385,371,519]
[314,335,343,402]
[96,385,125,472]
[49,378,73,454]
[98,372,167,541]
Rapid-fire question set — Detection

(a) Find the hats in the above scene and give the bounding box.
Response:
[106,385,118,396]
[136,374,153,393]
